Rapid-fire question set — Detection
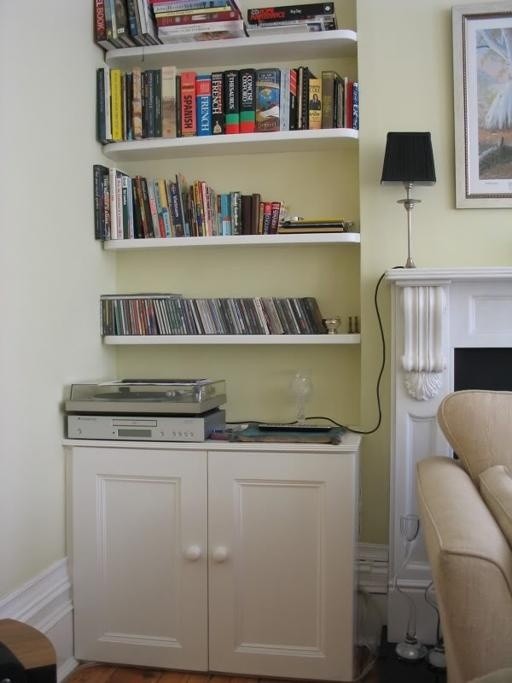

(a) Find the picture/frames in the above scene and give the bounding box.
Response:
[452,0,512,209]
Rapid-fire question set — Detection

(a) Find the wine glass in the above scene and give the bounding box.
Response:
[322,318,341,334]
[287,376,313,426]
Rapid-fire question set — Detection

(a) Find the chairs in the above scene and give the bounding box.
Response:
[415,391,512,683]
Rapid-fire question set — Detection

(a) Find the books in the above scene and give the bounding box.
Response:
[94,0,339,51]
[229,424,346,444]
[100,294,327,336]
[93,164,353,241]
[97,65,360,147]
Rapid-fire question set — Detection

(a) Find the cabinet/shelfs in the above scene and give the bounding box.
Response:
[61,426,363,682]
[102,29,359,343]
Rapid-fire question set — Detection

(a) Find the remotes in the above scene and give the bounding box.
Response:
[258,422,332,431]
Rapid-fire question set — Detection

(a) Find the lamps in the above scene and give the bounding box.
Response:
[380,132,436,268]
